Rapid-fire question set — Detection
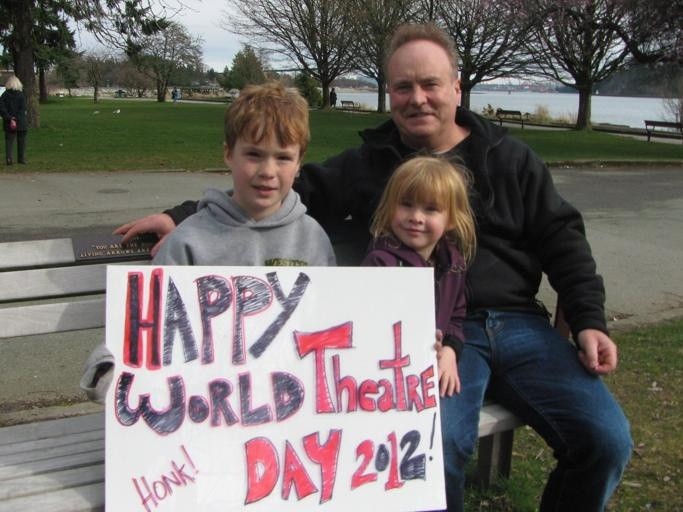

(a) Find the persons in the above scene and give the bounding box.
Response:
[0,74,30,166]
[354,148,483,402]
[75,77,341,414]
[109,18,636,512]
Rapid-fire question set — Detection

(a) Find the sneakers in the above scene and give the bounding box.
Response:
[7,158,28,165]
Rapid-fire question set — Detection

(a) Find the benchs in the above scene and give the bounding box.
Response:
[340,100,361,112]
[1,228,529,511]
[645,120,683,142]
[496,109,529,129]
